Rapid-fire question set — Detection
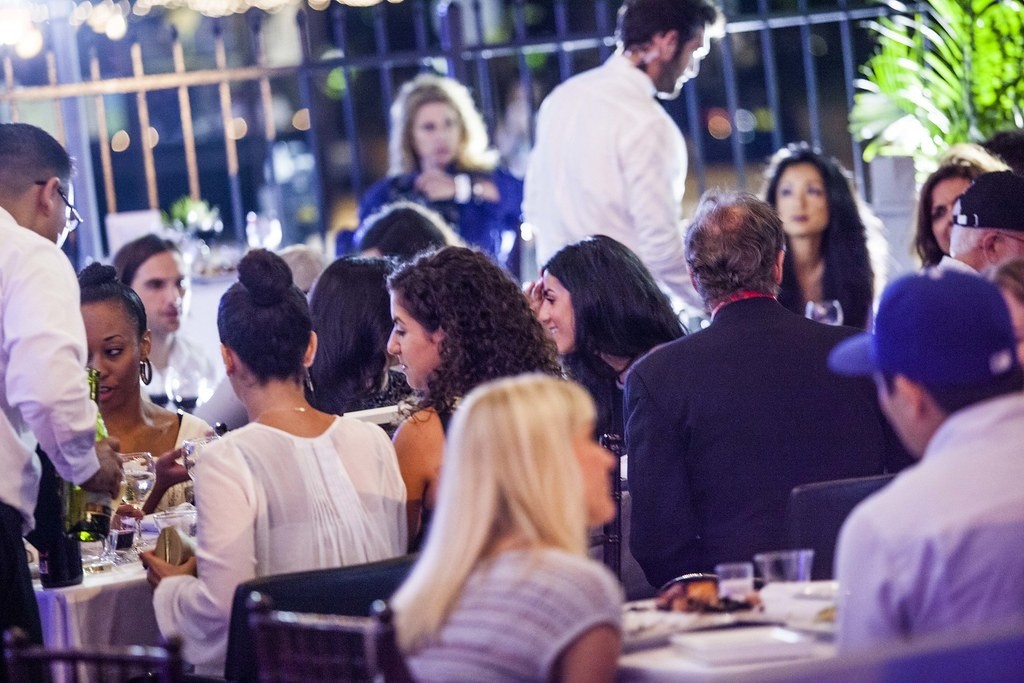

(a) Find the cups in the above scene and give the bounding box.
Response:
[245,212,282,252]
[754,548,814,587]
[152,511,192,539]
[717,563,754,612]
[182,437,219,482]
[79,529,118,575]
[803,298,844,326]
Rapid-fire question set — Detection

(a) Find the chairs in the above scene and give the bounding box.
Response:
[3,472,895,683]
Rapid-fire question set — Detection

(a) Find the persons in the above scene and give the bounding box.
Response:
[756,140,890,339]
[521,232,689,492]
[359,74,524,268]
[374,371,626,683]
[353,200,448,262]
[309,253,419,442]
[520,0,730,333]
[79,262,222,531]
[111,234,249,437]
[138,249,408,682]
[273,244,328,298]
[625,185,924,598]
[915,166,1024,369]
[0,122,124,683]
[828,267,1024,652]
[386,242,565,556]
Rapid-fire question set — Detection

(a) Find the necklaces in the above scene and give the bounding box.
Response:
[253,404,311,422]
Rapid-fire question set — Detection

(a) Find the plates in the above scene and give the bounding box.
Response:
[667,625,814,664]
[759,578,839,635]
[140,512,163,532]
[195,269,240,285]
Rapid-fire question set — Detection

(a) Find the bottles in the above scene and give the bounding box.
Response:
[60,365,115,542]
[31,444,83,589]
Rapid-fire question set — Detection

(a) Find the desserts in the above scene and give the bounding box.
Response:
[657,573,753,612]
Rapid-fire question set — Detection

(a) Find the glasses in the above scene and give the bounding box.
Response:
[30,181,82,234]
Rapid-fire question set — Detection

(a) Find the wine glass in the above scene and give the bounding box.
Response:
[118,451,156,548]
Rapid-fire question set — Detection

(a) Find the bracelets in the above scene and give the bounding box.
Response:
[470,176,483,205]
[455,174,471,202]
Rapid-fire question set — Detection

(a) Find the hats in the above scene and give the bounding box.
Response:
[951,169,1024,233]
[825,274,1018,388]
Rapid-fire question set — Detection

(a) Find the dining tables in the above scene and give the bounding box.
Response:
[620,579,831,683]
[25,511,193,683]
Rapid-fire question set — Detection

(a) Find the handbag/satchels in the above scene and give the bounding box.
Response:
[154,525,197,568]
